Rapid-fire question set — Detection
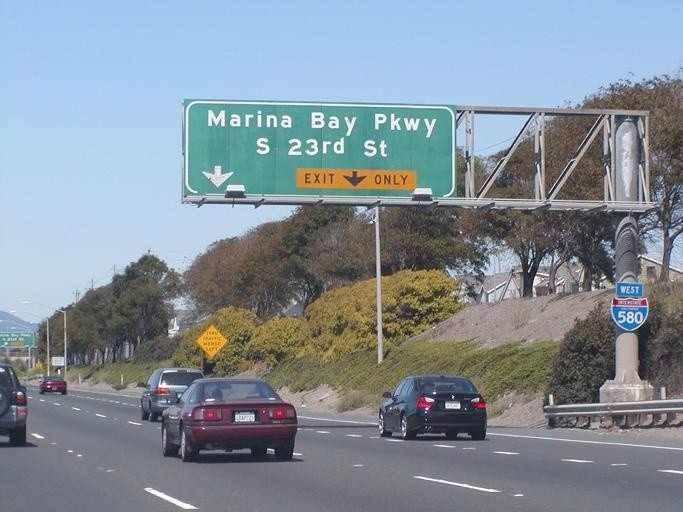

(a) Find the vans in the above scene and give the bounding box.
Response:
[141,369,208,426]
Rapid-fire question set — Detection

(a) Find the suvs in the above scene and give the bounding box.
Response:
[0,364,28,447]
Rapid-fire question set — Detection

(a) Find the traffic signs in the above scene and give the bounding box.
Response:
[176,99,454,199]
[610,298,649,332]
[0,333,35,348]
[616,282,645,299]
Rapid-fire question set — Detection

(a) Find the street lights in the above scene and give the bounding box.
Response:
[0,299,69,379]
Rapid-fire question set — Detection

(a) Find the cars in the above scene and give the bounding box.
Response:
[161,378,299,463]
[377,376,488,441]
[40,376,66,395]
